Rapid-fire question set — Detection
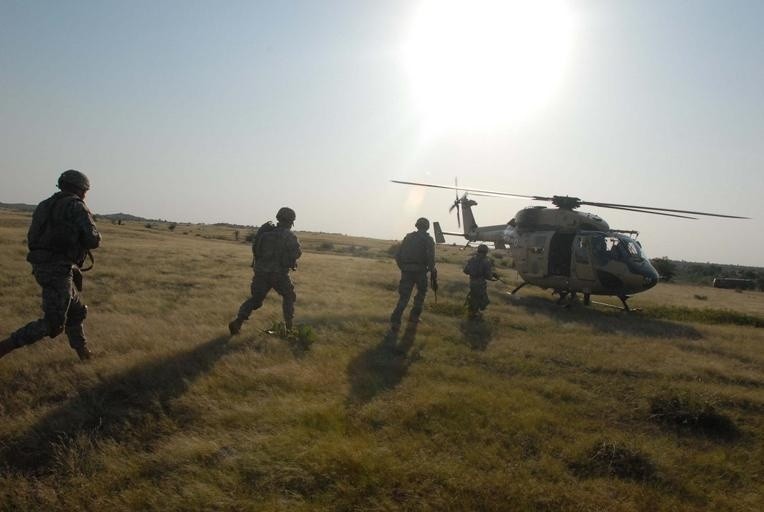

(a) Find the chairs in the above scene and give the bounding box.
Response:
[598,241,611,256]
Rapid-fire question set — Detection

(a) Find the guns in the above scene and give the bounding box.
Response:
[491,272,505,284]
[427,264,444,304]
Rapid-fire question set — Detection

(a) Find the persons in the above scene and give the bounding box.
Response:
[228,207,303,336]
[462,244,495,319]
[0,169,103,361]
[389,217,437,333]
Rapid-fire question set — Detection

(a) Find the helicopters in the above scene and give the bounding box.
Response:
[391,176,750,312]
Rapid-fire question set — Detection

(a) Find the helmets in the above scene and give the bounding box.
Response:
[477,245,489,254]
[416,218,429,230]
[59,170,90,194]
[276,207,296,222]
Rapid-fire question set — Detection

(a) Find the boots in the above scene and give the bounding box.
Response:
[286,319,294,330]
[229,315,244,335]
[0,337,15,358]
[76,343,109,361]
